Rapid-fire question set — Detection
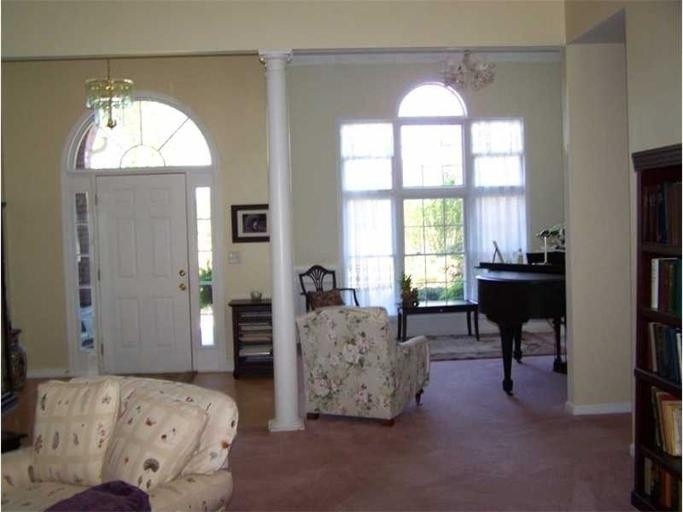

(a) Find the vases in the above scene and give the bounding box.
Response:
[8,329,26,390]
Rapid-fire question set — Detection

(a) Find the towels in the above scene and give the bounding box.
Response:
[44,480,151,512]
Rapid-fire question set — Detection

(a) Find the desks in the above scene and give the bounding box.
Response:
[396,297,480,341]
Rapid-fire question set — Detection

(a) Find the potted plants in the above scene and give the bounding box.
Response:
[399,271,412,304]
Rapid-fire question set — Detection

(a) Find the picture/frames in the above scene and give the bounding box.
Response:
[229,203,269,242]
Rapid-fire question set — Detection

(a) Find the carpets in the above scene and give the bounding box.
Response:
[395,329,566,360]
[116,371,198,383]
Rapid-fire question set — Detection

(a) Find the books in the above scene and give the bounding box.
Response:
[237,310,272,365]
[634,170,682,510]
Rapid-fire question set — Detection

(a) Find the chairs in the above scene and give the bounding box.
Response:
[298,265,360,313]
[1,372,243,512]
[296,304,429,426]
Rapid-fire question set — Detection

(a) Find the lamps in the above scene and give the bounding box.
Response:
[439,50,496,93]
[84,57,135,129]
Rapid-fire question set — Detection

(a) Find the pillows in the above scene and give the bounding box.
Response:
[100,389,211,494]
[31,377,122,486]
[309,289,346,309]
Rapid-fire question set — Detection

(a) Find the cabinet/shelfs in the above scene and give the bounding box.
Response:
[625,141,682,511]
[227,297,272,380]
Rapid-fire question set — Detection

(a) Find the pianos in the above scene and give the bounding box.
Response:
[475,263,565,393]
